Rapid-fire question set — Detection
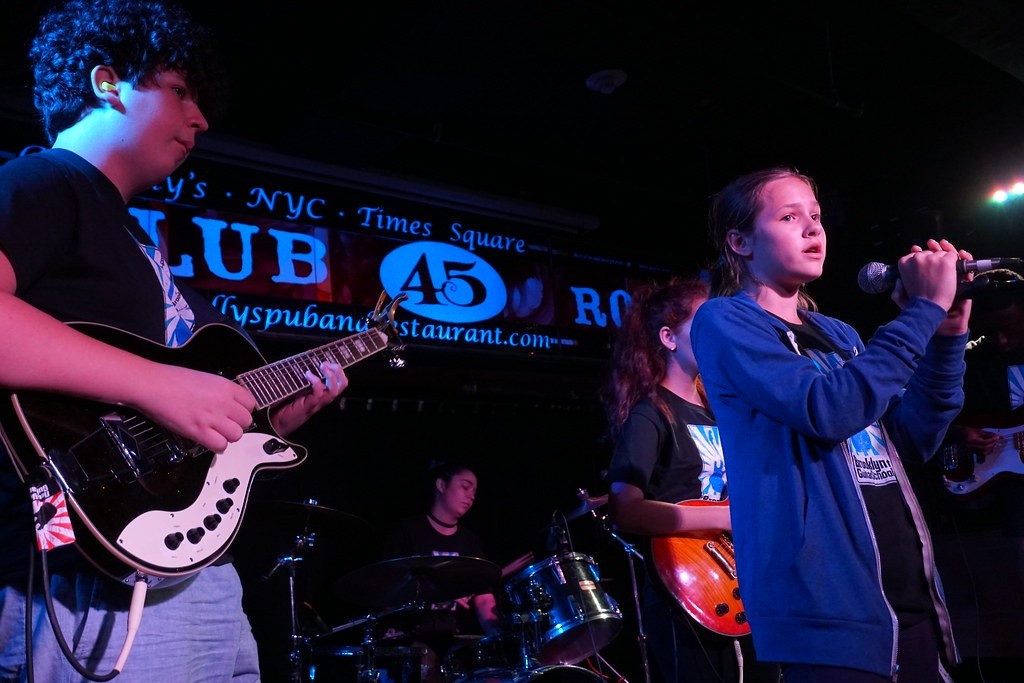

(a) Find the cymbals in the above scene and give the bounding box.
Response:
[337,552,503,608]
[539,486,609,535]
[270,496,361,521]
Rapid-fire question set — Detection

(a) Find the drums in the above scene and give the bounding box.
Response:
[517,664,607,683]
[438,631,536,683]
[505,551,624,666]
[306,643,424,683]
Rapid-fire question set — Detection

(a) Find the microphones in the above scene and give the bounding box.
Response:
[546,512,561,551]
[857,257,1020,295]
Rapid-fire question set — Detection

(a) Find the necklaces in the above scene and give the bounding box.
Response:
[428,512,457,528]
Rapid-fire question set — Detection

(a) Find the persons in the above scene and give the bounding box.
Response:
[608,167,1024,683]
[0,0,349,683]
[364,455,499,667]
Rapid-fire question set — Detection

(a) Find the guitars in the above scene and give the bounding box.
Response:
[0,285,412,592]
[938,421,1024,503]
[647,494,754,643]
